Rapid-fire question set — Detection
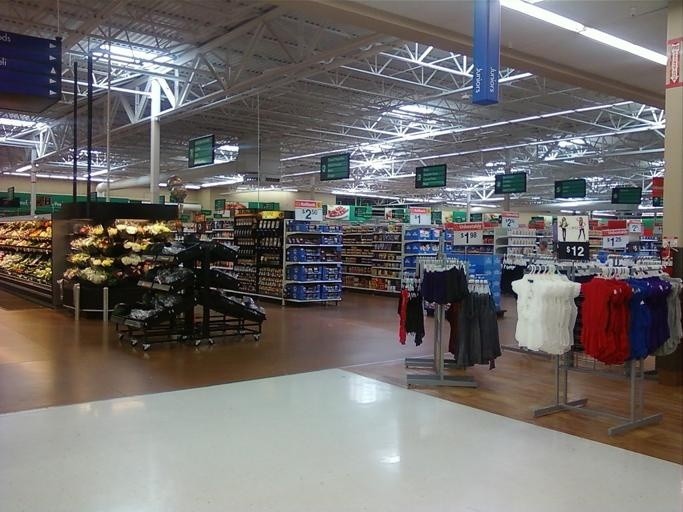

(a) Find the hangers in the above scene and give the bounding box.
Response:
[660,257,676,277]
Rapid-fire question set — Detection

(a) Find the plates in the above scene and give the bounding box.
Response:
[325,210,349,220]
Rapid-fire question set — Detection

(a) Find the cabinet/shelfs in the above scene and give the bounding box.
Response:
[0,203,661,350]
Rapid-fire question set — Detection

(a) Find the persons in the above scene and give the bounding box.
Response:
[576,217,585,239]
[559,217,568,241]
[538,241,550,256]
